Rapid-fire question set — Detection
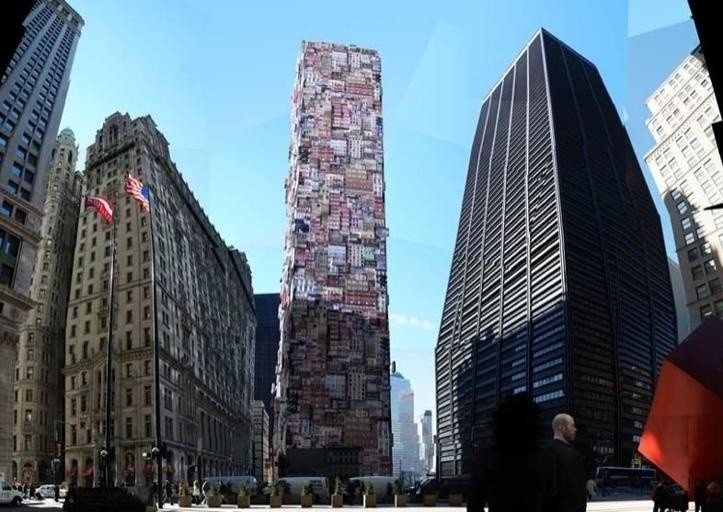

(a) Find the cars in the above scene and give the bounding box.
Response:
[0,481,68,507]
[408,476,456,503]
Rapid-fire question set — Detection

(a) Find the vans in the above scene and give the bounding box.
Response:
[263,476,331,504]
[342,476,399,505]
[187,476,258,503]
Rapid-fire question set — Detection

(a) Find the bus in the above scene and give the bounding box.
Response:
[594,466,657,490]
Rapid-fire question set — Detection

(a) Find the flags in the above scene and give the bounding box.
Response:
[86,196,114,224]
[125,174,149,212]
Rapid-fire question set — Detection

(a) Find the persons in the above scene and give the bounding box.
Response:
[651,480,723,512]
[163,480,229,505]
[538,413,587,512]
[54,483,60,502]
[11,481,36,500]
